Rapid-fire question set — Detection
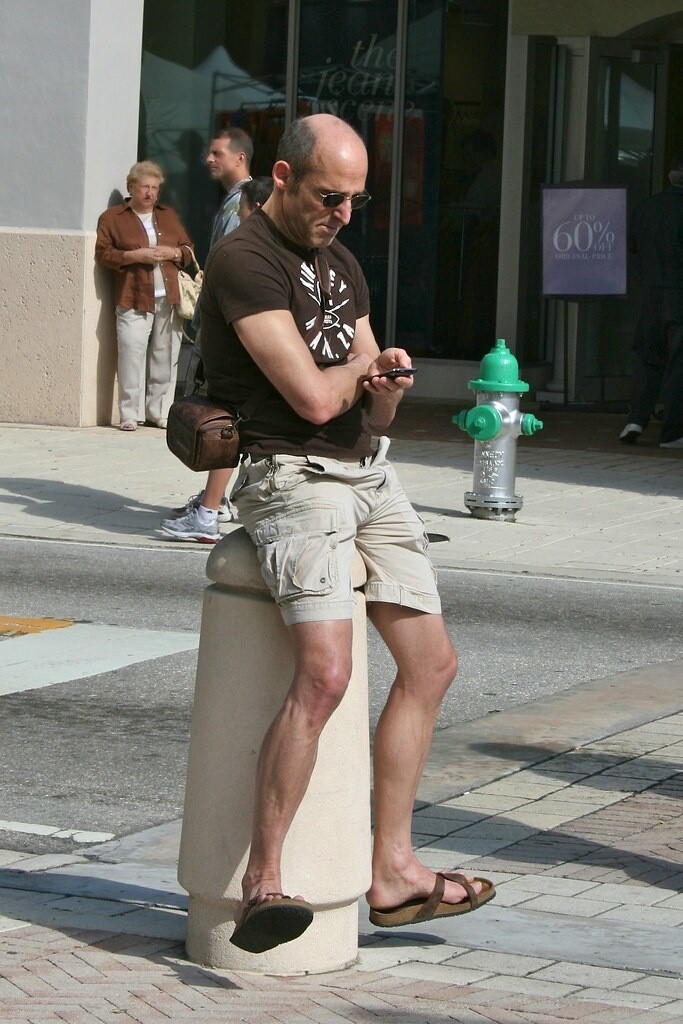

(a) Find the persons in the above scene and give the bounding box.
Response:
[189,113,495,954]
[618,151,683,449]
[95,161,193,430]
[161,127,274,543]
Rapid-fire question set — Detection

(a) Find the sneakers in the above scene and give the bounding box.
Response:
[171,490,233,523]
[159,509,221,542]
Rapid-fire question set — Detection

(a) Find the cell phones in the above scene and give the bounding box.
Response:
[362,369,418,385]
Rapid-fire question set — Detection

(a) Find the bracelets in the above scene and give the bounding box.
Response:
[173,248,178,262]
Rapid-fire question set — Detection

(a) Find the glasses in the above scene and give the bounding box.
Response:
[300,175,371,209]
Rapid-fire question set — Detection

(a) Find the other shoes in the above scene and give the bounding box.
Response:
[119,422,137,430]
[143,418,166,428]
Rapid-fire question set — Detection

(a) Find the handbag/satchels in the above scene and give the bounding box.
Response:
[177,245,205,320]
[166,394,241,473]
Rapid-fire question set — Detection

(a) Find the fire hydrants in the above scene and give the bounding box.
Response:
[451,338,544,522]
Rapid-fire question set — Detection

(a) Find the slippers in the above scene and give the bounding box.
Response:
[369,872,498,929]
[229,891,314,953]
